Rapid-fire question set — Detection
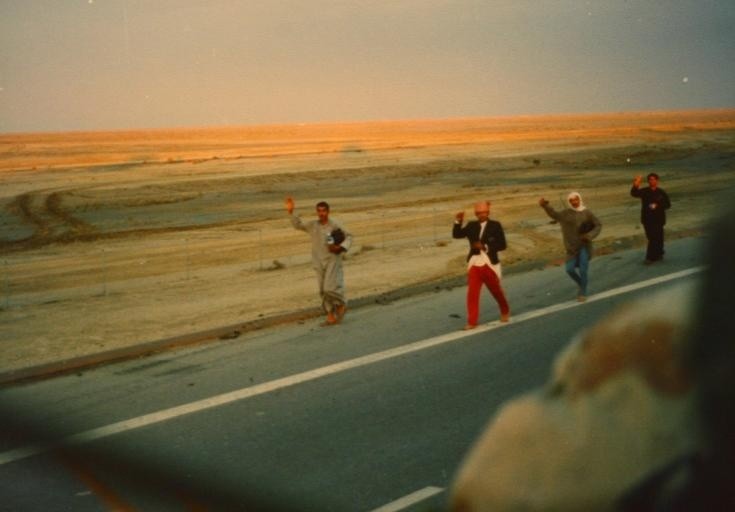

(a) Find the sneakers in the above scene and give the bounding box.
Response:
[464,324,479,330]
[577,295,587,302]
[500,314,508,322]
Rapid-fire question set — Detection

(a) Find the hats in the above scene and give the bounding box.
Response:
[475,201,490,213]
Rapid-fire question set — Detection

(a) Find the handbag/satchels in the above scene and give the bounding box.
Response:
[331,227,344,243]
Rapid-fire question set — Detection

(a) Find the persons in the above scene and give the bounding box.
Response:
[538,191,603,302]
[451,200,510,330]
[284,195,353,327]
[630,172,672,266]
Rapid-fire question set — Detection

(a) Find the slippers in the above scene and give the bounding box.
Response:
[320,306,348,328]
